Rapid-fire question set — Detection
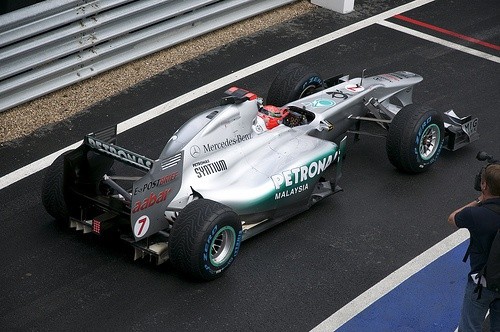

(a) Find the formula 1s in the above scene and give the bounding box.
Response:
[41,62,480,284]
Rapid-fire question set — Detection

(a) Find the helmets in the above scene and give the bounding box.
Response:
[258,105,282,130]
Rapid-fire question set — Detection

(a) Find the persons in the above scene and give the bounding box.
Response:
[255,105,290,131]
[449,164,500,332]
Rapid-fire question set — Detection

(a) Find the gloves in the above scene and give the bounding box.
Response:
[279,108,289,121]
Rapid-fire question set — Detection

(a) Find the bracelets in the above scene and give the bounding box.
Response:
[474,198,482,205]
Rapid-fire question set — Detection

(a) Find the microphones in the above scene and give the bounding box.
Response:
[476,152,493,161]
